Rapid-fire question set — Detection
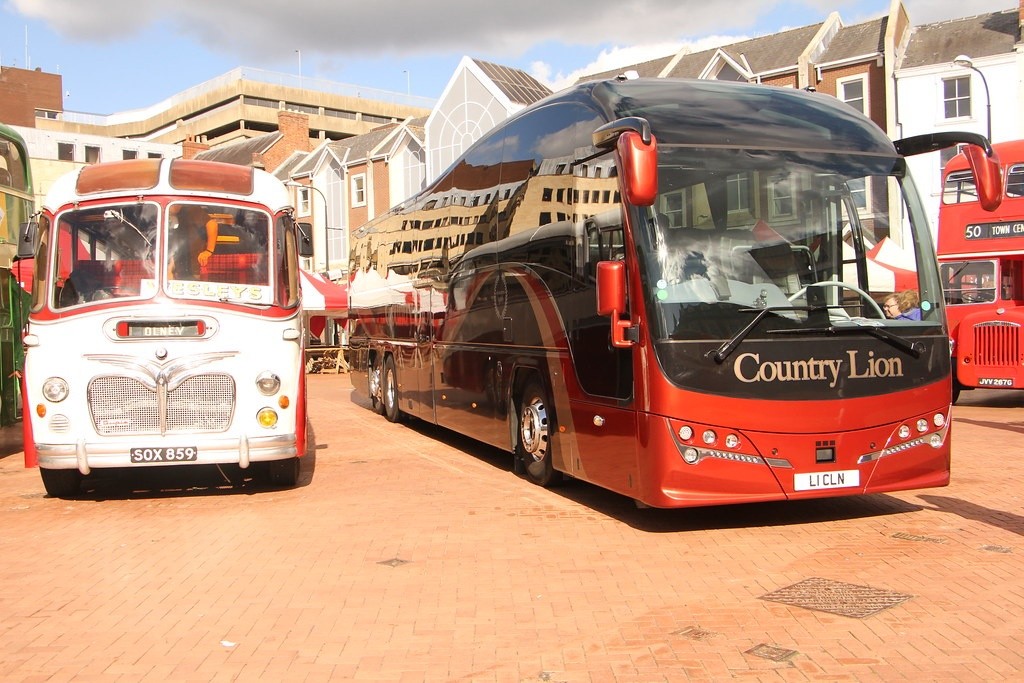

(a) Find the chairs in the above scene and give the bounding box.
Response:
[74,258,153,294]
[202,253,269,286]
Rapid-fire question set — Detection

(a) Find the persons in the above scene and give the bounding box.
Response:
[883,290,921,321]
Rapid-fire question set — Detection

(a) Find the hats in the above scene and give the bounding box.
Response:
[648,212,670,227]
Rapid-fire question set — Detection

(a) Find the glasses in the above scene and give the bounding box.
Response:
[882,304,894,311]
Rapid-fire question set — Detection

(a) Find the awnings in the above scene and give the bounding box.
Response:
[351,266,451,338]
[299,268,349,309]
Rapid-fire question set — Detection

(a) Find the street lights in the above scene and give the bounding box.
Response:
[282,180,329,348]
[954,54,991,140]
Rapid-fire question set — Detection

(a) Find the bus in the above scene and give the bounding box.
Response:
[349,76,1002,516]
[18,158,315,498]
[936,140,1024,404]
[0,123,48,420]
